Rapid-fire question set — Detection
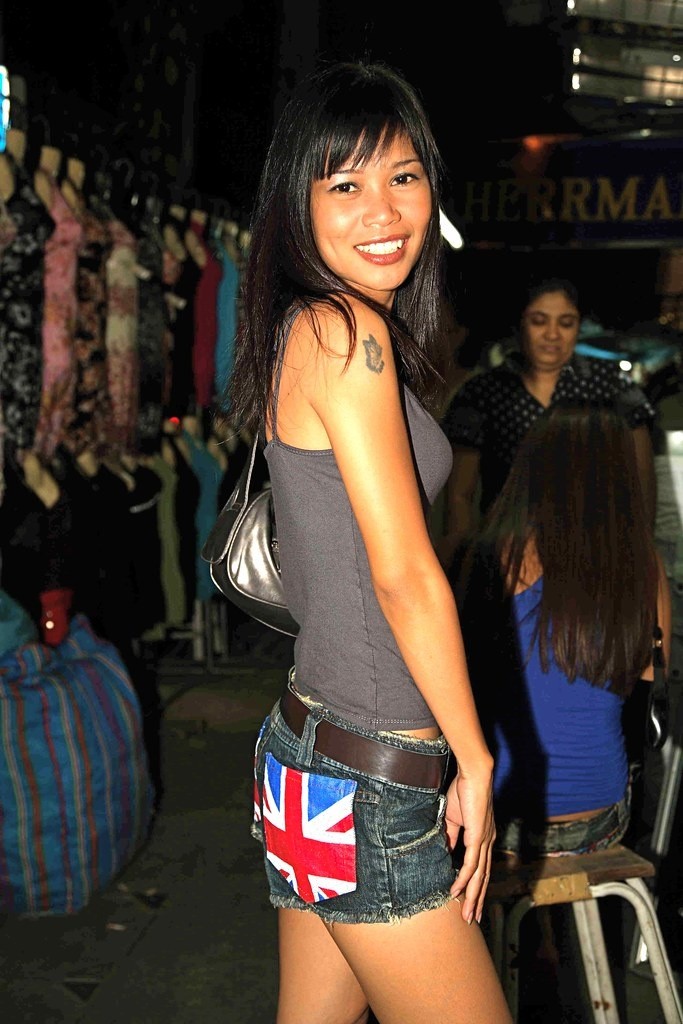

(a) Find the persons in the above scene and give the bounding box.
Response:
[222,62,683,1024]
[0,129,253,508]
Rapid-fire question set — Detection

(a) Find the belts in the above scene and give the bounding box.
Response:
[279,685,452,789]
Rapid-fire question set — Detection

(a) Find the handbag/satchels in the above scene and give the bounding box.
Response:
[0,614,153,914]
[201,424,301,638]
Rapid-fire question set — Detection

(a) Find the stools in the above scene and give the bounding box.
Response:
[450,844,683,1024]
